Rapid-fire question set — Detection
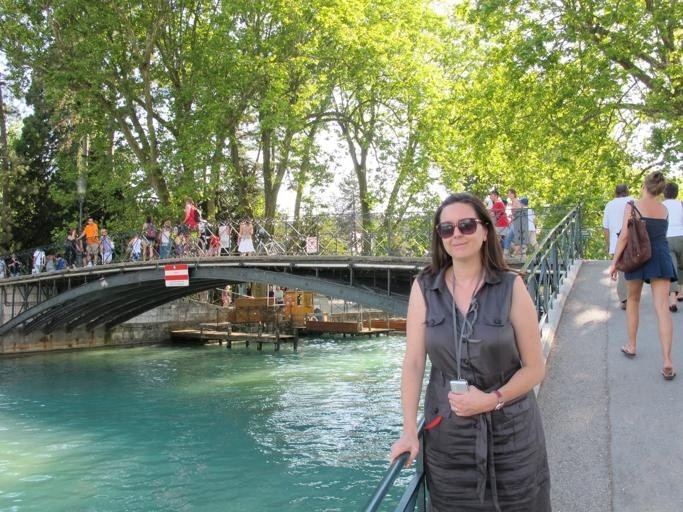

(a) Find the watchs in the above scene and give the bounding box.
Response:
[491,390,504,411]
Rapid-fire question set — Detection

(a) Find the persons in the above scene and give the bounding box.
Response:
[389,193,552,512]
[662,182,683,311]
[603,184,639,310]
[488,188,541,258]
[0,198,284,307]
[609,171,679,380]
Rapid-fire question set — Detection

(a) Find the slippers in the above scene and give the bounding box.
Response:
[621,345,634,356]
[661,369,675,380]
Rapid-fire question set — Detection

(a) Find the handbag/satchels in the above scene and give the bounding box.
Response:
[616,219,650,272]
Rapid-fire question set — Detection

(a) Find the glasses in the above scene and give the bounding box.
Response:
[435,218,488,238]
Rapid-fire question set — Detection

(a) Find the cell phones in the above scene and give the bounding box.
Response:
[450,380,469,393]
